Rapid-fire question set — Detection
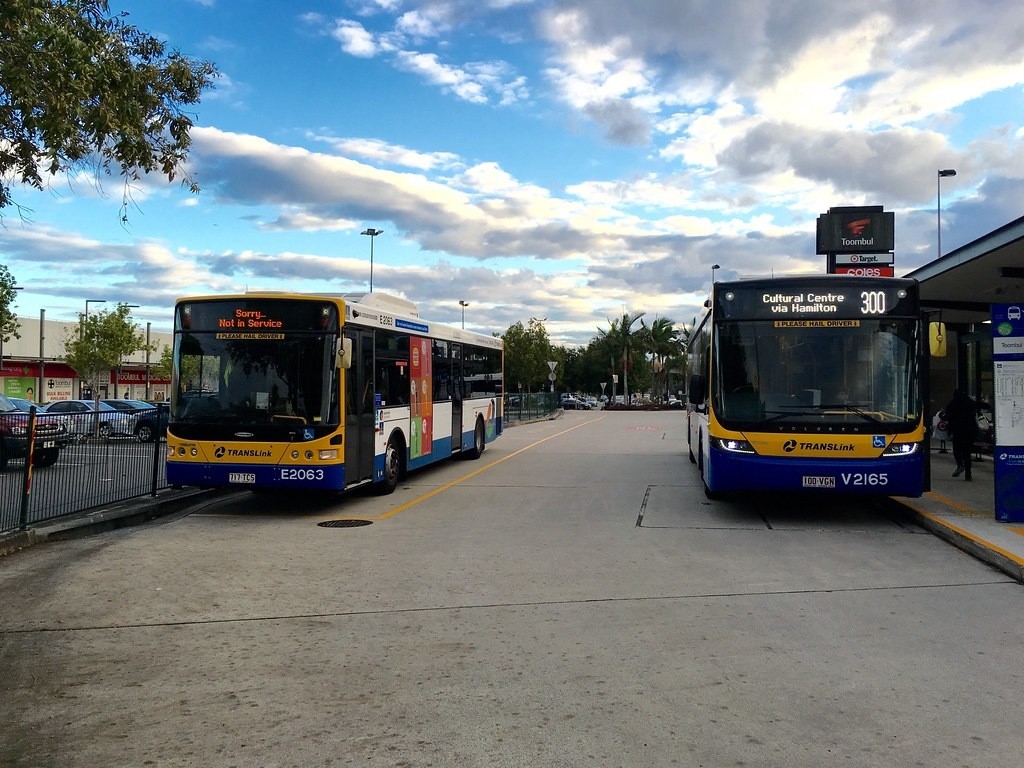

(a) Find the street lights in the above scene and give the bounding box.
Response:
[360,224,384,296]
[711,264,721,286]
[458,298,469,325]
[936,168,959,258]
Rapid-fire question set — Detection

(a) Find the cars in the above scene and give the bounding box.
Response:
[7,395,170,443]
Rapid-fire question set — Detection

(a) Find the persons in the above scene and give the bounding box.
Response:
[124,388,129,399]
[26,387,33,401]
[933,389,989,481]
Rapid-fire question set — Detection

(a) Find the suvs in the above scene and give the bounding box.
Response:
[1,392,66,471]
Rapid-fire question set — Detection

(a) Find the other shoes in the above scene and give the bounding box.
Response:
[952,466,964,477]
[965,475,972,481]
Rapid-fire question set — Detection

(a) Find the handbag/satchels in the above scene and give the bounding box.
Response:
[932,411,952,442]
[976,415,990,431]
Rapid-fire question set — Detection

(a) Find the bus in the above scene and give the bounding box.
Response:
[158,287,511,506]
[682,268,951,505]
